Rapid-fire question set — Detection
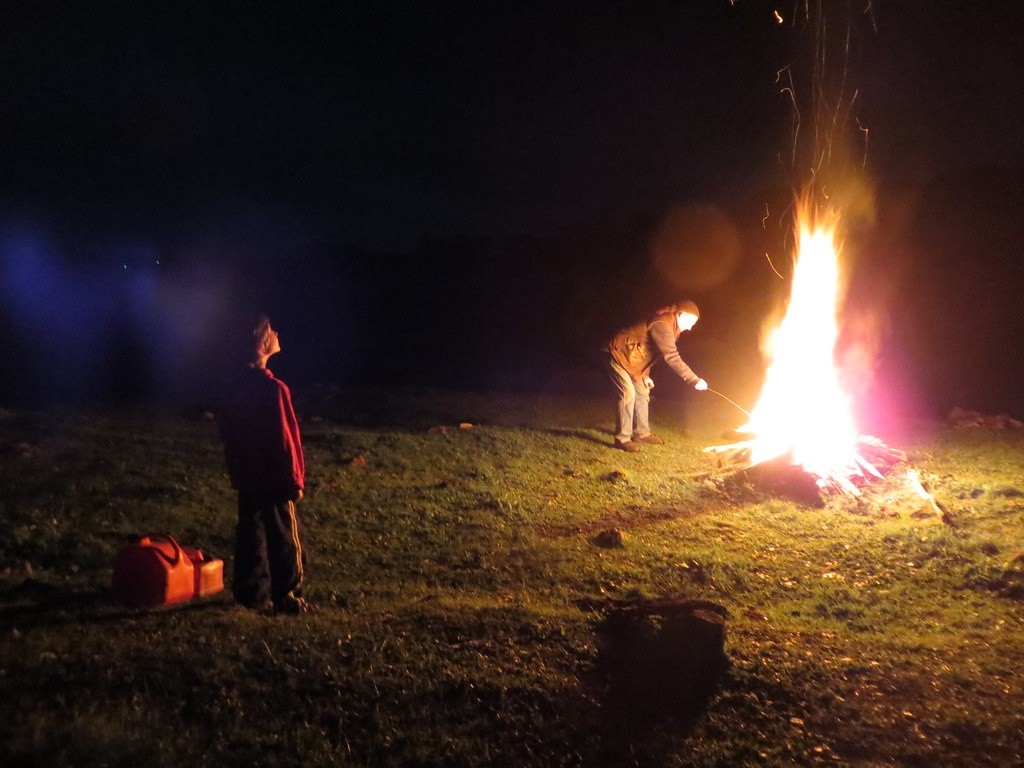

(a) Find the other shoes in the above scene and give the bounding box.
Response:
[614,438,640,452]
[276,595,319,616]
[250,600,273,614]
[632,435,664,445]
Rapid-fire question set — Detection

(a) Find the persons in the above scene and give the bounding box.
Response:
[216,311,306,612]
[600,300,708,452]
